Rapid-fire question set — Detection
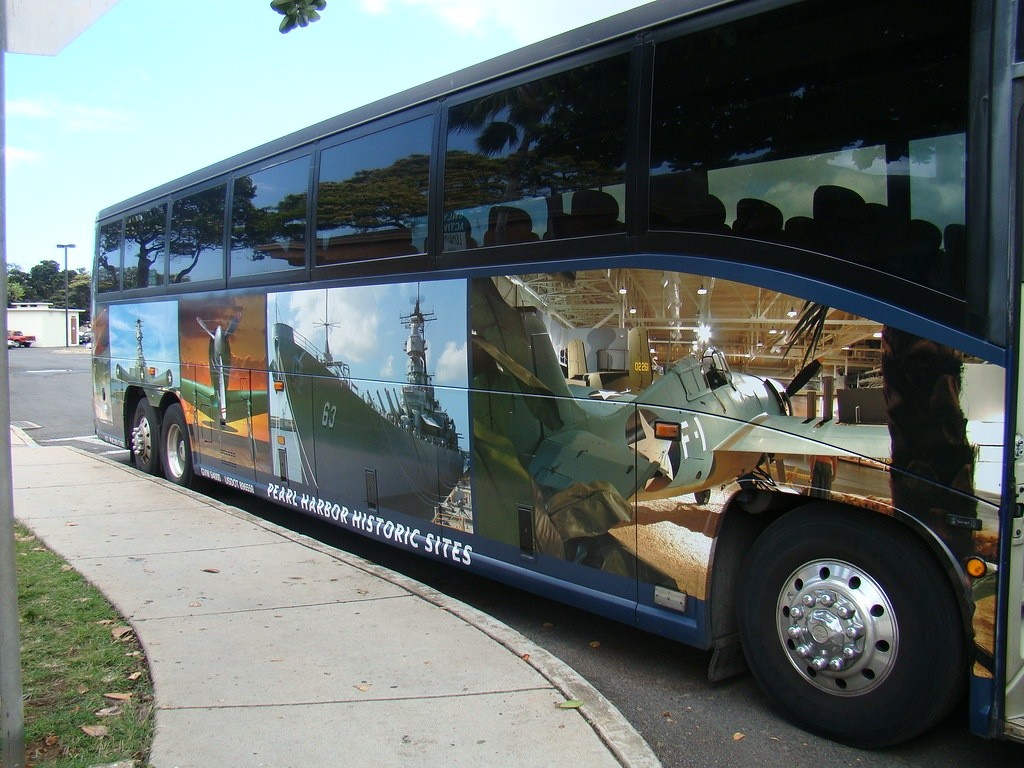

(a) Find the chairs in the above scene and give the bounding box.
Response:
[252,187,976,299]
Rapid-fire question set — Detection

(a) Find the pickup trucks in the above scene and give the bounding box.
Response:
[8,330,36,348]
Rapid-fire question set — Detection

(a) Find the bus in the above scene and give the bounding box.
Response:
[88,0,1023,754]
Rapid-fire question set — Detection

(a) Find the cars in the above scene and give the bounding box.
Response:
[8,340,15,349]
[79,321,92,345]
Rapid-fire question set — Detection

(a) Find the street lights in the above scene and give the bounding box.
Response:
[56,244,76,347]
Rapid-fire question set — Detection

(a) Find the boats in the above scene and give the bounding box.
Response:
[272,283,466,523]
[108,316,181,406]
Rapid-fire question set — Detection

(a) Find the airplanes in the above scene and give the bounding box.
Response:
[180,311,268,432]
[469,277,893,516]
